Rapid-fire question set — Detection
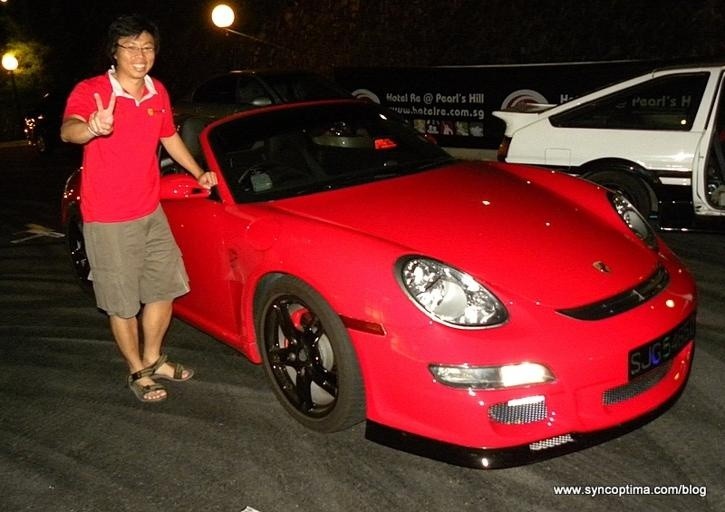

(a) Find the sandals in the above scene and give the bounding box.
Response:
[150,352,194,382]
[128,367,168,403]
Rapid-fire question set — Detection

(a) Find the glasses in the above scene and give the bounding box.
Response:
[117,43,156,55]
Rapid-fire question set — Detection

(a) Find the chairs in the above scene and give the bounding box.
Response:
[176,114,240,201]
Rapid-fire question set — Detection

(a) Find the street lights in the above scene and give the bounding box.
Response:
[2,55,25,125]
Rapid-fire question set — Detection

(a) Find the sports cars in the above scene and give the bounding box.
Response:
[493,65,724,231]
[62,97,698,470]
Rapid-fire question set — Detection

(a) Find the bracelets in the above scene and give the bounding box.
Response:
[87,127,99,137]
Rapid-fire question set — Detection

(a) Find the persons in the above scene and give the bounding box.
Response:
[61,9,219,404]
